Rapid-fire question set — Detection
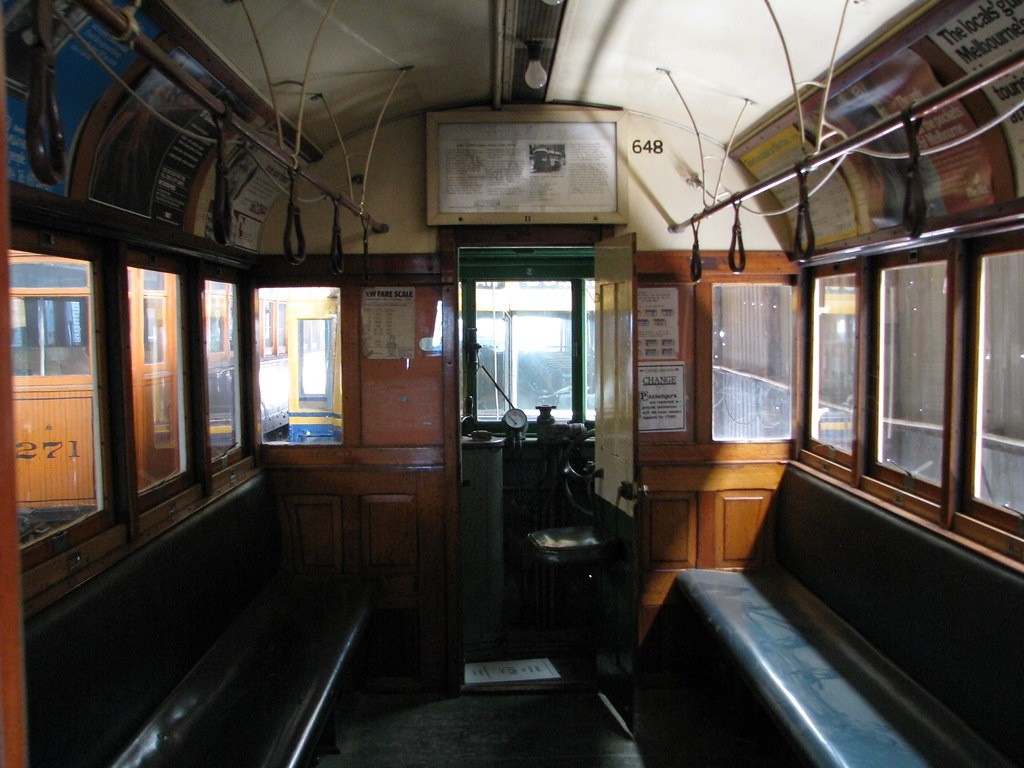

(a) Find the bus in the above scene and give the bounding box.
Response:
[288,281,594,445]
[7,247,323,526]
[715,278,899,459]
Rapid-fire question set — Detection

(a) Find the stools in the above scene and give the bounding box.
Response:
[526,524,620,671]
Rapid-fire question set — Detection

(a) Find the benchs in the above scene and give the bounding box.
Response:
[22,465,379,768]
[676,460,1024,768]
